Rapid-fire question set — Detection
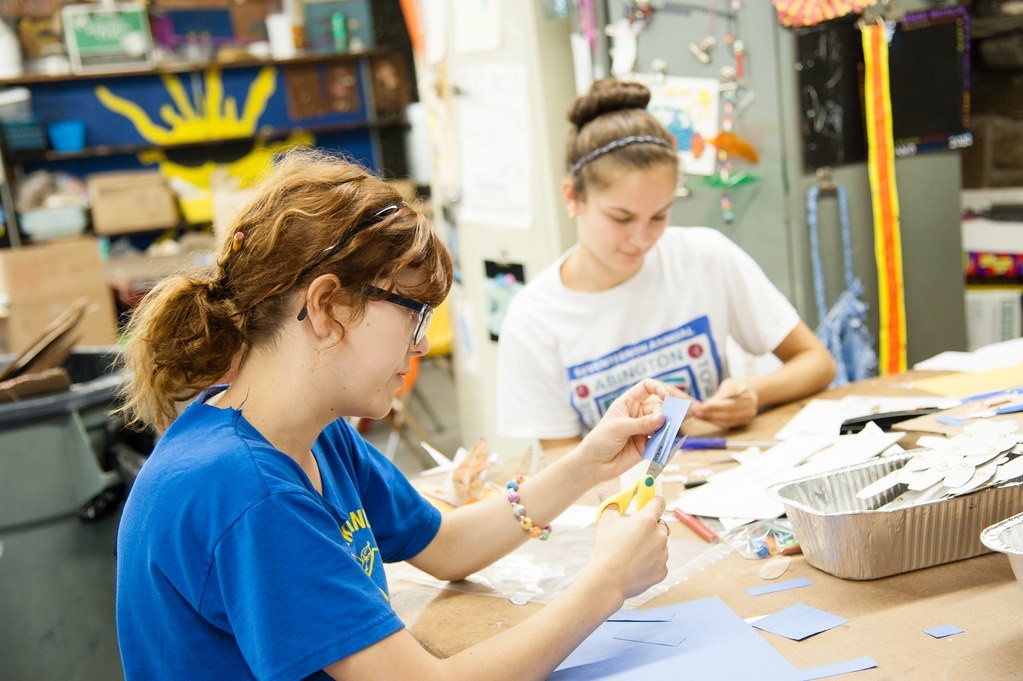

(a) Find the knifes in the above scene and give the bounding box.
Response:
[674,436,786,450]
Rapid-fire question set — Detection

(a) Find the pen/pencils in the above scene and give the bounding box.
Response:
[674,436,769,449]
[674,508,717,543]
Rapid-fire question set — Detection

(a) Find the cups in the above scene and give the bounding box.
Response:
[266,14,306,59]
[49,120,85,154]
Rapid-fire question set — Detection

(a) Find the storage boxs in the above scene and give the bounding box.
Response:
[0,232,120,355]
[86,170,181,236]
[766,445,1023,582]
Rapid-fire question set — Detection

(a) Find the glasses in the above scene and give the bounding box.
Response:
[297,278,434,347]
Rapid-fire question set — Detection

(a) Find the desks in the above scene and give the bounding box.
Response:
[384,367,1023,681]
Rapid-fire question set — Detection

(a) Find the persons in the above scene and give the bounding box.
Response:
[497,81,836,450]
[116,151,669,681]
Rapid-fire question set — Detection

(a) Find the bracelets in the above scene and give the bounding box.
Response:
[506,475,552,541]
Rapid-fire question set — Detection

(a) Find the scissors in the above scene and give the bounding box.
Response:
[593,421,690,530]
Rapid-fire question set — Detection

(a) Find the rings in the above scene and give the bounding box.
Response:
[657,519,670,536]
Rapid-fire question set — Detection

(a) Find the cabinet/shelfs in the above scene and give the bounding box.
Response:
[0,44,400,247]
